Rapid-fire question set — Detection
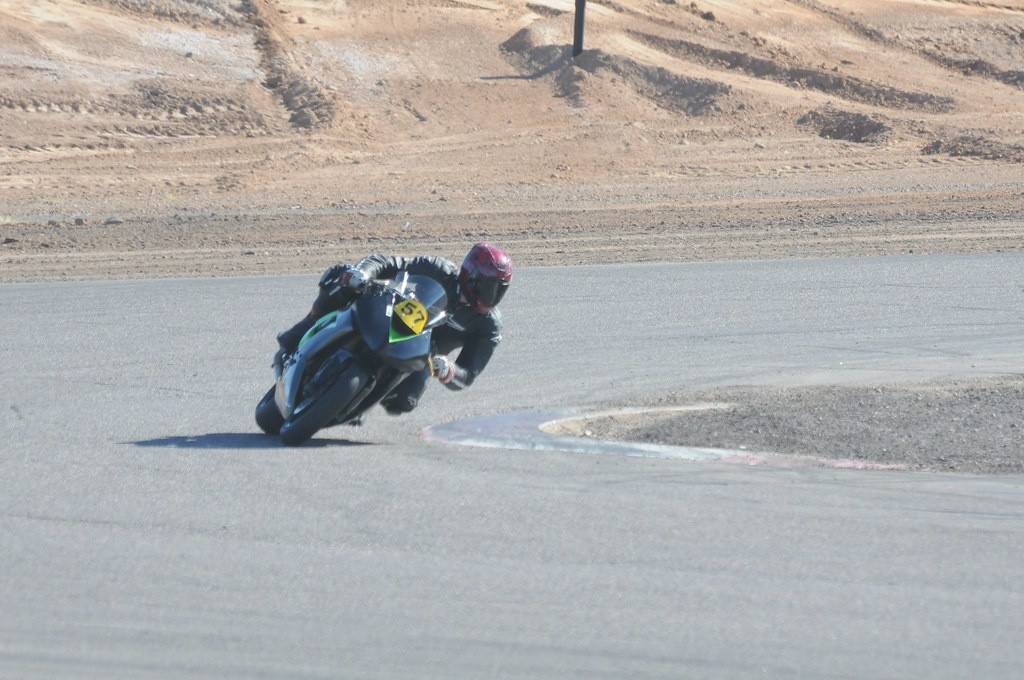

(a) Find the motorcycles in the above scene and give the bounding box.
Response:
[255,270,447,444]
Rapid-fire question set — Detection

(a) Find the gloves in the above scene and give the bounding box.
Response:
[341,268,368,290]
[430,354,449,379]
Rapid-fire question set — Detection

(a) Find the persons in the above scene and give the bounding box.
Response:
[276,241,514,417]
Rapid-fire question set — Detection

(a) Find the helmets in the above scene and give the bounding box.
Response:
[458,241,512,307]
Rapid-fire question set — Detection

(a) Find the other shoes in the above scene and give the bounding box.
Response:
[384,395,415,416]
[277,322,306,351]
